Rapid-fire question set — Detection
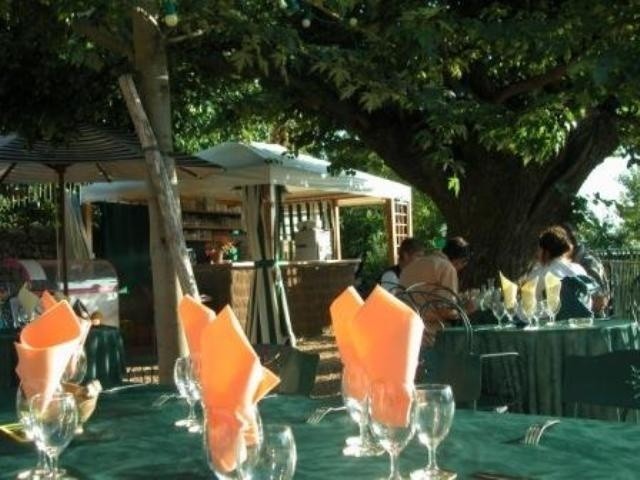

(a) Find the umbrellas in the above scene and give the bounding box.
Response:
[0,114,225,301]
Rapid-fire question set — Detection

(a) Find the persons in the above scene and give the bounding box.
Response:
[375,238,426,295]
[518,226,591,316]
[392,235,486,330]
[561,221,611,320]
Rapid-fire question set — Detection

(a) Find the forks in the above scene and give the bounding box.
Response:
[523,418,561,446]
[305,405,345,425]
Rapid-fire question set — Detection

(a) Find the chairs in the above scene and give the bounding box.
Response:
[246,342,321,397]
[352,274,640,411]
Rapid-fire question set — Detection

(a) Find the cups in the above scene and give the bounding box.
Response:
[198,404,299,480]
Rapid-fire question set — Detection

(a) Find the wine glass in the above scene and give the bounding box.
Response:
[490,300,564,331]
[171,356,205,435]
[16,381,79,480]
[337,372,460,479]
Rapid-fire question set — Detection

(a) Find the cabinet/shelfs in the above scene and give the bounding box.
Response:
[182,209,247,243]
